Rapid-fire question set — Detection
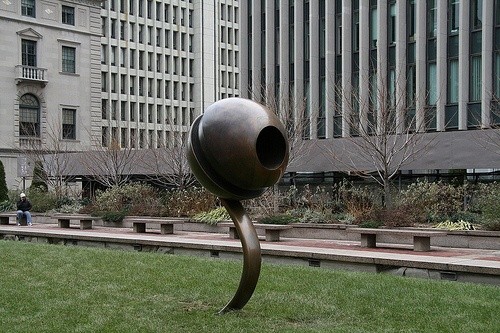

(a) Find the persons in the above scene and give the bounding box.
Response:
[16,193,32,226]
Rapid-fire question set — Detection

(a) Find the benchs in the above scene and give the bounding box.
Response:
[216,222,293,242]
[123,219,184,235]
[51,216,101,230]
[0,214,36,225]
[346,227,448,252]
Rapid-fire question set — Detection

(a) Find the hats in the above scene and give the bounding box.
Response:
[20,193,26,197]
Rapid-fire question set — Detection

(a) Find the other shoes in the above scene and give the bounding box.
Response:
[18,222,22,227]
[27,222,32,226]
